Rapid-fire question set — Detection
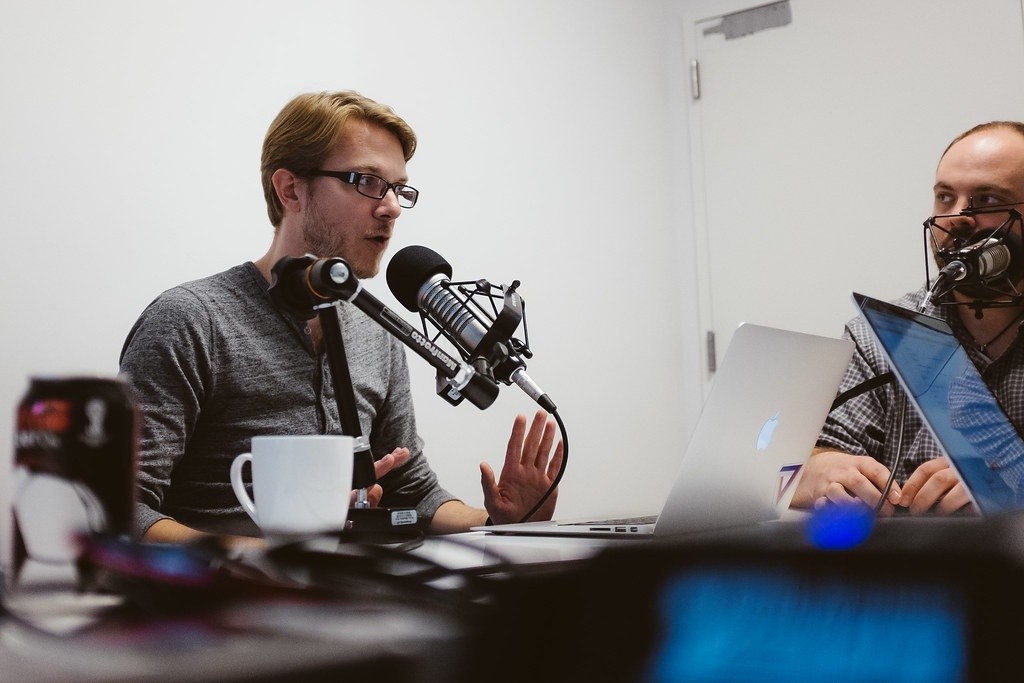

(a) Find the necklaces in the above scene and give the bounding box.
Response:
[956,311,1024,352]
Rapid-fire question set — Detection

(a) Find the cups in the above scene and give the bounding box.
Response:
[230,435,354,543]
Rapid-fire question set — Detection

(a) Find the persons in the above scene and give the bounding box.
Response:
[119,92,566,549]
[789,120,1024,515]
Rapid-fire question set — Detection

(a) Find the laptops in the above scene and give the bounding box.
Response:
[831,289,1024,527]
[469,322,861,537]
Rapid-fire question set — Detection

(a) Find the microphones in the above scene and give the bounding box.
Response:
[945,225,1023,282]
[383,245,528,389]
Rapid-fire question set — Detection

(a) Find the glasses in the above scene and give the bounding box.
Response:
[288,167,420,209]
